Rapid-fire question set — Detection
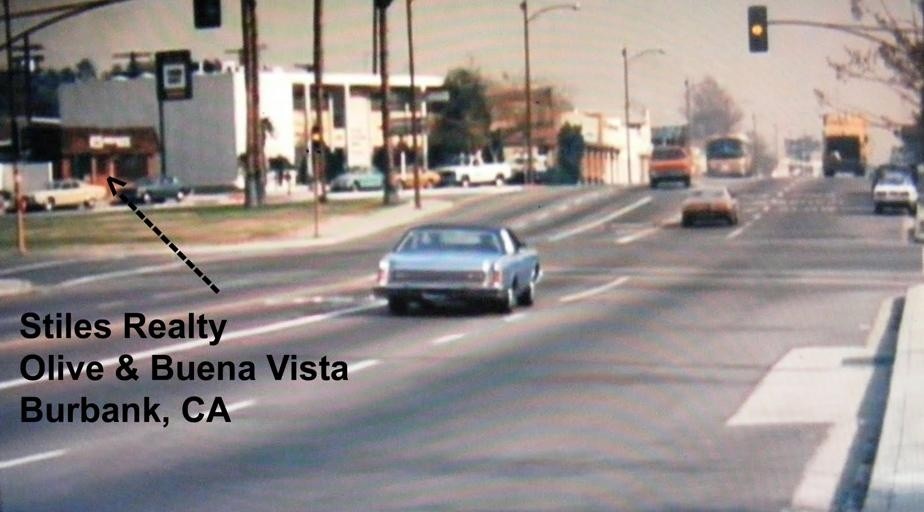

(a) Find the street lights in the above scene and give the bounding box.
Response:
[620,43,669,186]
[683,71,714,147]
[520,0,582,187]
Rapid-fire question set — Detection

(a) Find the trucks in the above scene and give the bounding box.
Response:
[821,113,868,167]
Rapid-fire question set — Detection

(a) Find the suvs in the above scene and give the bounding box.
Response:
[821,134,864,179]
[646,145,696,190]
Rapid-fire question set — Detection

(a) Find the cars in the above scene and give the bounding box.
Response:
[332,155,551,195]
[0,186,14,219]
[681,187,740,225]
[375,225,544,314]
[25,179,109,213]
[118,171,193,206]
[869,162,924,214]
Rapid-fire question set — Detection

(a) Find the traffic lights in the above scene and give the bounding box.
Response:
[748,5,766,51]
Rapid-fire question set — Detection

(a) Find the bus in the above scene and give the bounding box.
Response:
[702,131,755,176]
